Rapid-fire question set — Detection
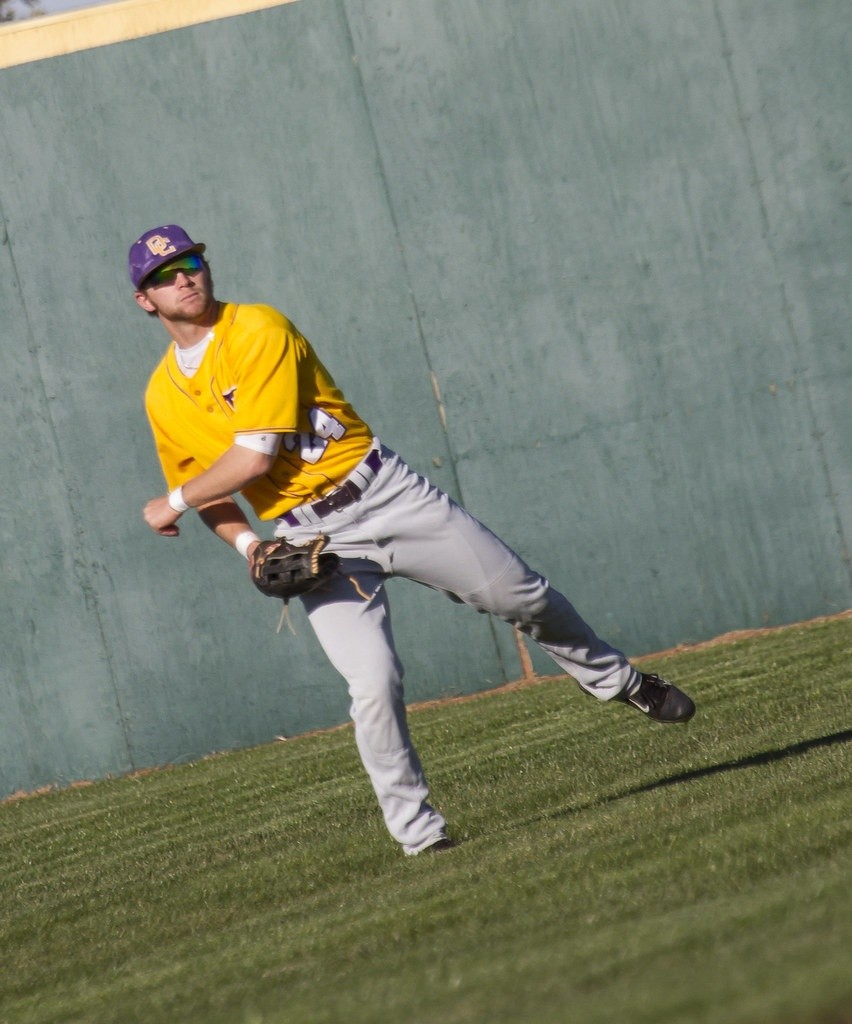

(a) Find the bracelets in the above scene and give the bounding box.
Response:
[168,483,190,514]
[234,531,262,563]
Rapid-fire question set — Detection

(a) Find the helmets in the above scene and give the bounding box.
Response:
[129,225,206,286]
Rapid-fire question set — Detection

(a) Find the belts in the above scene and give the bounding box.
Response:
[280,449,384,527]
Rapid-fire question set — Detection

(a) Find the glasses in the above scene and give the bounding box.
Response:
[141,254,201,288]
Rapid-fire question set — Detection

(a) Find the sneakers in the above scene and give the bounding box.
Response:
[578,671,697,724]
[420,838,458,857]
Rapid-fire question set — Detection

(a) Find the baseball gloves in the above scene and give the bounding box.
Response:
[251,534,341,598]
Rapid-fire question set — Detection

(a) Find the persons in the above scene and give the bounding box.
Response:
[127,224,698,866]
